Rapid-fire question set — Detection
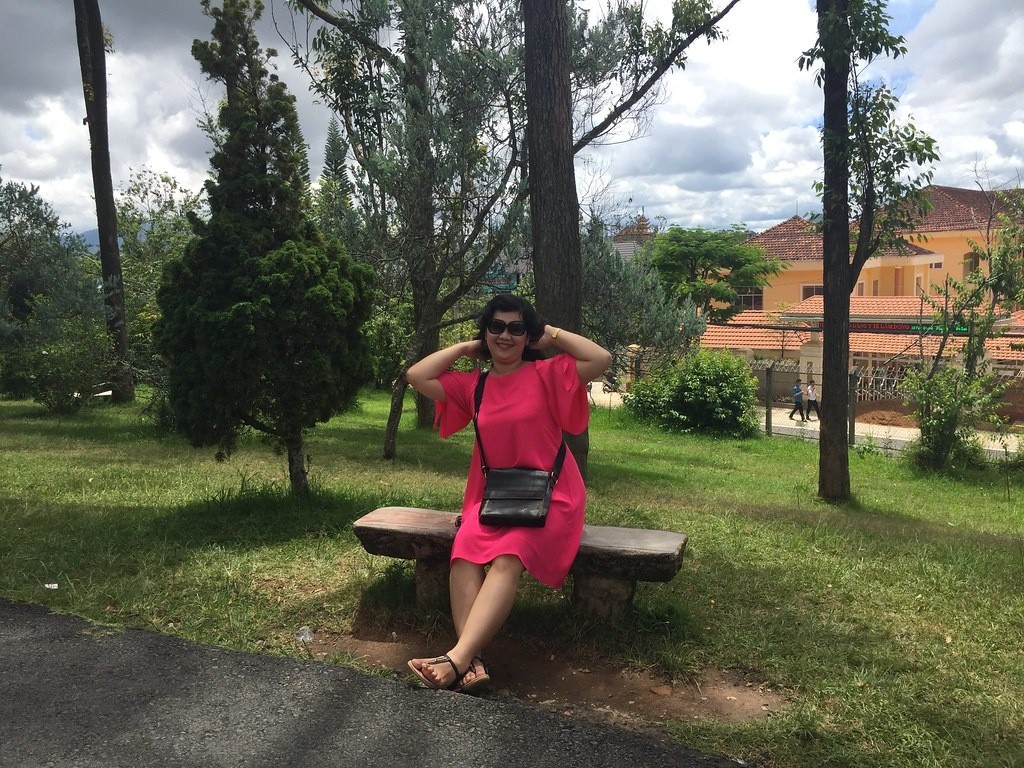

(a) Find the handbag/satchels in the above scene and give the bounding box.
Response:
[478,468,554,527]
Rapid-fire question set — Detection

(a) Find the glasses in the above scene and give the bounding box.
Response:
[487,319,526,336]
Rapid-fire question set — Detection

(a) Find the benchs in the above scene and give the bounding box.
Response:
[351,507,689,627]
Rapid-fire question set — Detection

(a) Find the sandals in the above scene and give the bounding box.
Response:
[459,656,490,692]
[408,654,463,688]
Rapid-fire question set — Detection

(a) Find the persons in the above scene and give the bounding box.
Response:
[788,378,807,421]
[806,380,823,420]
[406,293,612,690]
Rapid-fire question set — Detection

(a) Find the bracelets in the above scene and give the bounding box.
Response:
[552,327,561,345]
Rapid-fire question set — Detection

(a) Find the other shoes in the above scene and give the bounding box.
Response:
[802,419,807,422]
[789,416,794,420]
[806,416,811,420]
